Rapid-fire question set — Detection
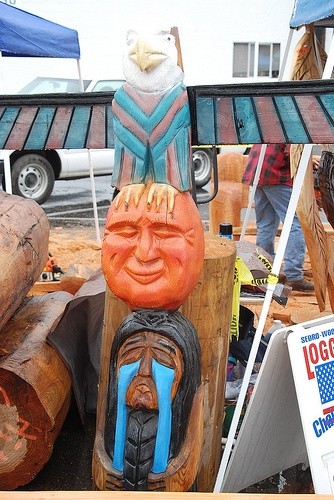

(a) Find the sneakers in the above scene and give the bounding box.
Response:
[278,271,287,283]
[285,276,314,292]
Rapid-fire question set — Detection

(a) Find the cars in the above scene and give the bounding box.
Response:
[0,72,215,204]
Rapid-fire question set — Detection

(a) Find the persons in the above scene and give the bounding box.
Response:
[222,304,285,438]
[241,143,316,295]
